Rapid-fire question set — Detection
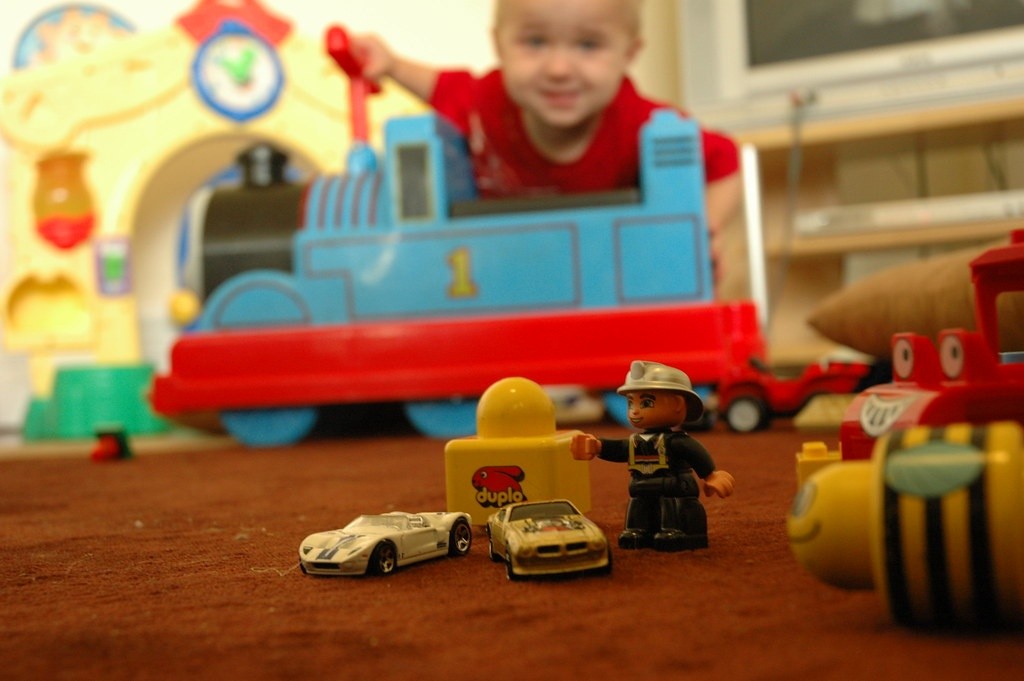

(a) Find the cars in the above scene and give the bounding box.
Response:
[484,498,614,583]
[296,509,473,579]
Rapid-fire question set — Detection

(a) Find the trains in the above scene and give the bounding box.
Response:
[144,105,766,451]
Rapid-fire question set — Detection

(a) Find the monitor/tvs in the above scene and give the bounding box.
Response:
[679,0,1024,132]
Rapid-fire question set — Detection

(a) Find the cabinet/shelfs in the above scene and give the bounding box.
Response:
[712,92,1024,368]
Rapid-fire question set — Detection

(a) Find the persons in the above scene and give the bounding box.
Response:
[359,0,743,297]
[569,360,733,550]
